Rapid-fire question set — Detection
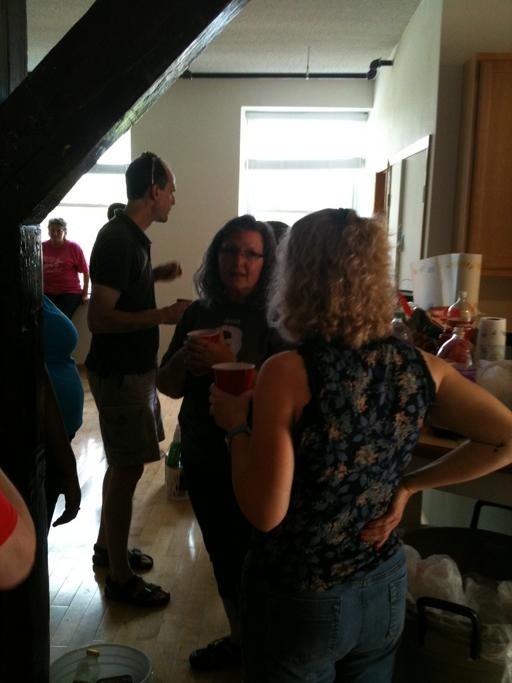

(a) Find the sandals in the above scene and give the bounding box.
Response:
[92,544,154,569]
[104,570,171,606]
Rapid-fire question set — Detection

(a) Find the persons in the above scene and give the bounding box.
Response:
[154,216,289,673]
[42,218,89,320]
[0,468,37,590]
[108,203,125,221]
[85,151,192,613]
[43,295,85,528]
[211,210,511,682]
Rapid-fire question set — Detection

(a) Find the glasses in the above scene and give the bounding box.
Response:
[239,248,262,261]
[142,152,157,185]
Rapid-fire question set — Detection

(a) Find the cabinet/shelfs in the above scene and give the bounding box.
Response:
[449,50,512,284]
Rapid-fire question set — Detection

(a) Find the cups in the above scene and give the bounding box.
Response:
[212,360,256,394]
[176,297,193,322]
[186,326,222,364]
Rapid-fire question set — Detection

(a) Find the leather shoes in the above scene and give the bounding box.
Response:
[189,633,237,670]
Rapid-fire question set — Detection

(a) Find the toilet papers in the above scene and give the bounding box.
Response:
[410,253,482,317]
[475,316,507,361]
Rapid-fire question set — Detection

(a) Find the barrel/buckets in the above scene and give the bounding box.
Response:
[392,496,512,682]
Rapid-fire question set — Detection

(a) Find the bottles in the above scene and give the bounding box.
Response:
[390,312,412,345]
[436,327,475,385]
[446,288,474,326]
[73,647,101,683]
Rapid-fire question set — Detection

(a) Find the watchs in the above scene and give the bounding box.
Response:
[225,426,250,448]
